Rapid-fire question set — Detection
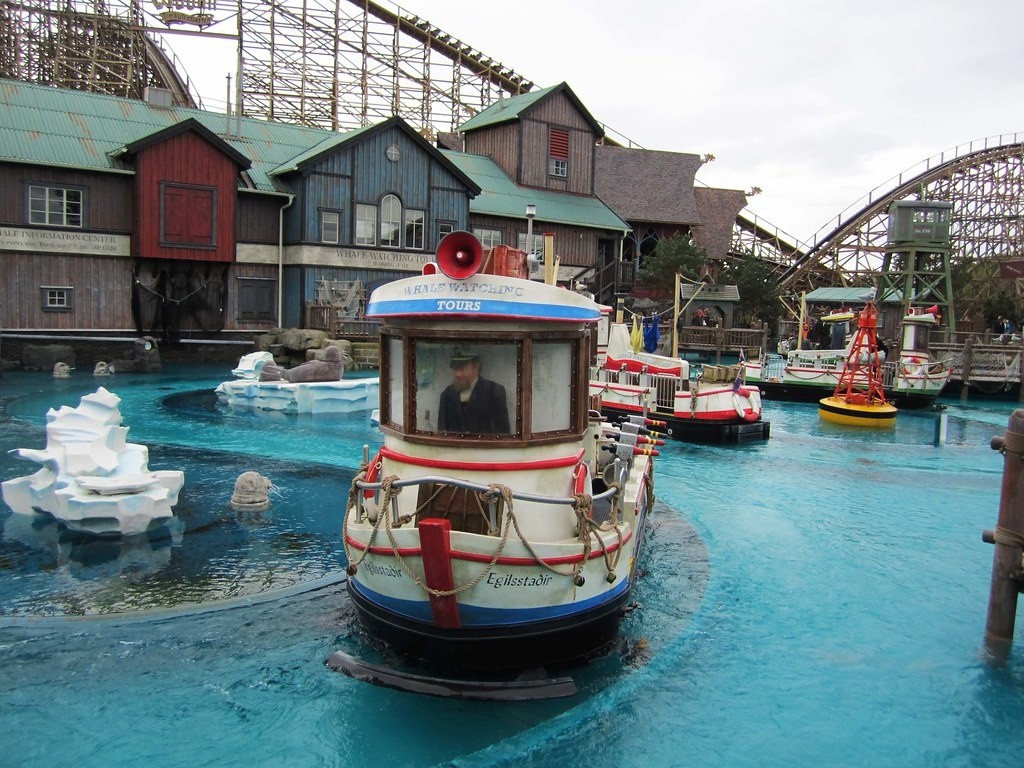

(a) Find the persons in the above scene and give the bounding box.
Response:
[438,343,510,434]
[802,324,810,339]
[993,315,1012,333]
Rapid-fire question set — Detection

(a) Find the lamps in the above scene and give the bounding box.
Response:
[525,204,536,217]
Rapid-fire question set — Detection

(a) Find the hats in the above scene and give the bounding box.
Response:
[448,343,480,368]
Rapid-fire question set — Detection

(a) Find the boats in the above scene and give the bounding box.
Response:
[733,281,955,408]
[325,203,669,704]
[587,297,773,446]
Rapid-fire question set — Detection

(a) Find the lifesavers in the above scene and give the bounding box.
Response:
[732,389,760,421]
[363,453,381,524]
[902,357,922,375]
[572,461,593,535]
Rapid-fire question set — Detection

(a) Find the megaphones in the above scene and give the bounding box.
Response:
[436,230,484,280]
[925,304,938,316]
